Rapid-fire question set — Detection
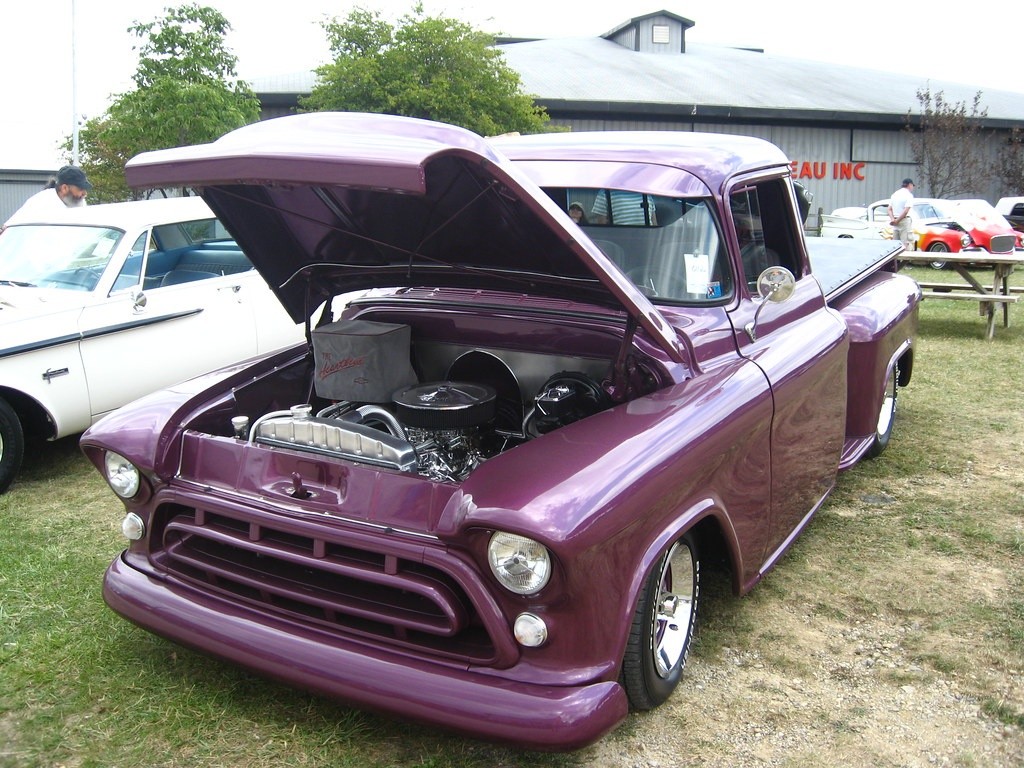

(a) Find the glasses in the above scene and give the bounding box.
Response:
[570,207,582,211]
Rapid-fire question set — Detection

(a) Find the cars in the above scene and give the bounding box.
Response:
[0,196,405,494]
[77,114,922,750]
[820,193,1024,270]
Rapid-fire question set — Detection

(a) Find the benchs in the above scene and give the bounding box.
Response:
[174,249,254,277]
[917,282,1024,316]
[922,292,1020,339]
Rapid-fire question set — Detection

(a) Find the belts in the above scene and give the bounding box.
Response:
[894,216,909,219]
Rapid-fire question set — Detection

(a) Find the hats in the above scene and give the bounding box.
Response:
[56,165,92,189]
[569,201,584,213]
[903,179,915,186]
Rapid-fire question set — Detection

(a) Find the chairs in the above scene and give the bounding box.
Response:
[654,240,720,286]
[590,238,627,271]
[159,269,221,287]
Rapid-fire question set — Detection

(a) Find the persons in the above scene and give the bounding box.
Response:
[887,178,915,251]
[0,166,93,233]
[591,188,658,226]
[568,201,591,226]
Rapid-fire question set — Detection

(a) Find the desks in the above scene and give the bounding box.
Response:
[894,251,1024,328]
